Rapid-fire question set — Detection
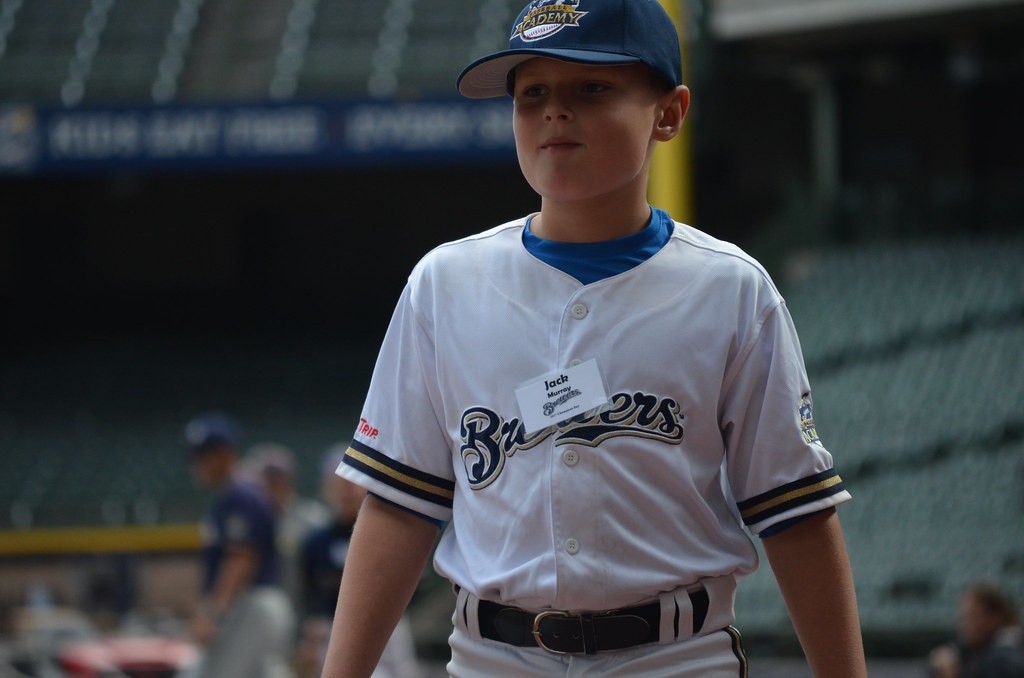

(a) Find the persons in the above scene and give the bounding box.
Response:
[0,535,208,677]
[185,413,416,678]
[320,0,870,678]
[926,576,1024,678]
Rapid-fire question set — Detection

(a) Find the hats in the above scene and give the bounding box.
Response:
[455,0,684,99]
[182,409,237,451]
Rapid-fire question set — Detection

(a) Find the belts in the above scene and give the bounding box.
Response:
[453,583,709,657]
